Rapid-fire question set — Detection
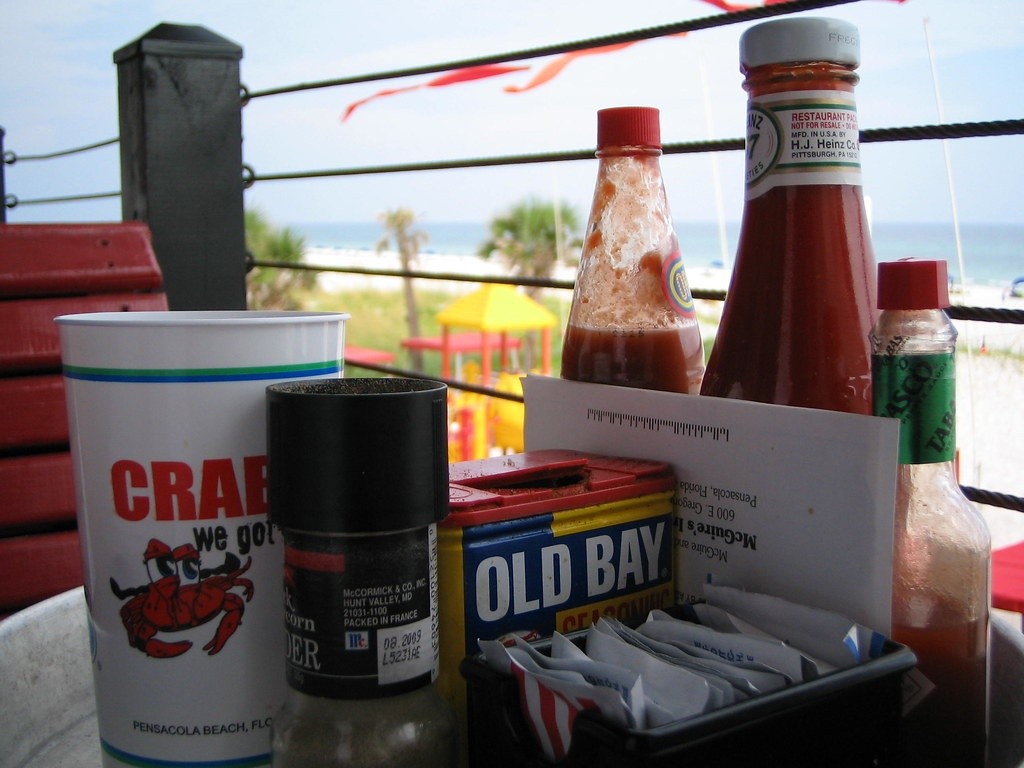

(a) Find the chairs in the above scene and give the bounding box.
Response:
[0,220,167,618]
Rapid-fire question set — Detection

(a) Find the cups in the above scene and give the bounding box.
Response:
[59,304,351,768]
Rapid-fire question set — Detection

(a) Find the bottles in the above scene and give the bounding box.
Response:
[559,106,705,400]
[858,259,988,720]
[703,16,883,414]
[260,377,456,768]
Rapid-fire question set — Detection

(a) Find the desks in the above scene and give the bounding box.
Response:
[3,580,105,767]
